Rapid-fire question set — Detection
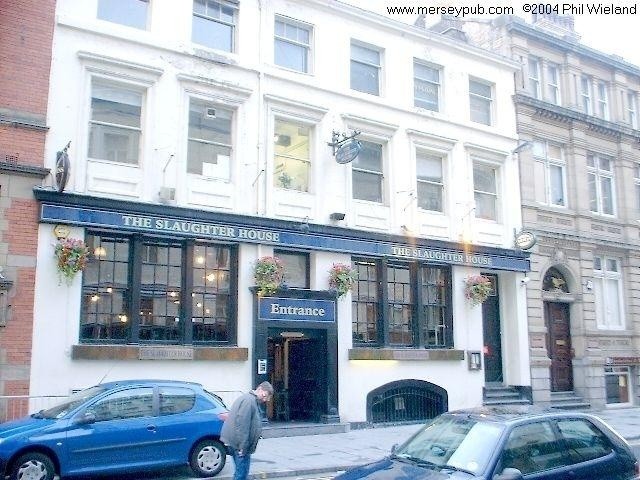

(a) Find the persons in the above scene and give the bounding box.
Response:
[216,381,275,479]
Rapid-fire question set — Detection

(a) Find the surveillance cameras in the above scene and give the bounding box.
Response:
[519,277,530,285]
[587,281,592,290]
[333,213,345,220]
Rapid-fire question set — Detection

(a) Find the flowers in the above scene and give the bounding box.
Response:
[327,261,359,299]
[252,255,288,298]
[463,274,492,307]
[54,236,91,287]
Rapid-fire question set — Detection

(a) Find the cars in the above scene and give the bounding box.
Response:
[1,377,234,479]
[333,403,640,480]
